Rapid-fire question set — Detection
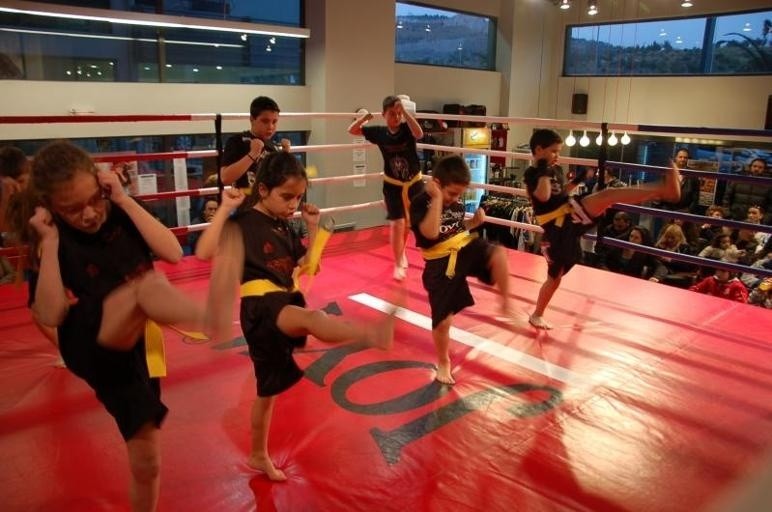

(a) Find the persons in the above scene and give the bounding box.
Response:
[701,204,732,235]
[219,95,292,186]
[738,204,765,245]
[189,195,219,225]
[21,143,210,511]
[524,128,684,332]
[407,155,517,385]
[673,148,700,213]
[620,226,668,282]
[673,216,683,225]
[345,92,434,286]
[698,234,746,263]
[346,94,424,281]
[593,166,624,195]
[598,207,635,263]
[688,266,749,304]
[113,162,133,195]
[194,148,400,482]
[653,222,691,262]
[746,277,772,309]
[742,254,772,287]
[748,157,768,178]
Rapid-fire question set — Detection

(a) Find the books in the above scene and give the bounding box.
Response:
[137,173,158,195]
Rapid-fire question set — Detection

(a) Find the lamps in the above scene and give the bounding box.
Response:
[424,24,430,32]
[585,0,600,17]
[457,42,463,51]
[607,130,618,147]
[659,27,667,37]
[396,21,403,29]
[742,20,752,32]
[595,130,603,146]
[270,37,276,44]
[267,45,272,51]
[559,0,572,12]
[680,0,695,8]
[564,129,577,147]
[579,130,591,148]
[675,36,682,45]
[242,33,248,41]
[620,131,630,145]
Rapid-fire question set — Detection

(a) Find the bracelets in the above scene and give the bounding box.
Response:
[239,148,264,167]
